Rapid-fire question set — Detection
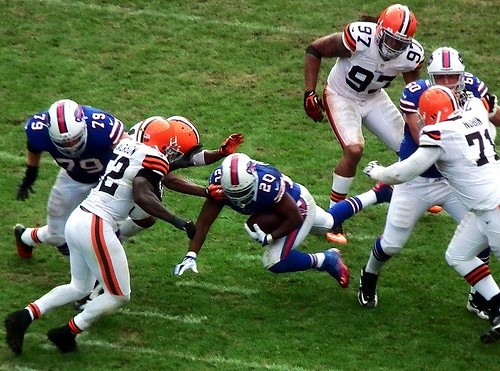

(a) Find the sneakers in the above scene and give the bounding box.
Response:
[357,266,378,307]
[375,181,394,203]
[56,243,70,255]
[326,249,350,288]
[481,316,500,344]
[46,324,77,352]
[466,292,490,320]
[13,224,32,260]
[4,308,32,355]
[324,227,348,245]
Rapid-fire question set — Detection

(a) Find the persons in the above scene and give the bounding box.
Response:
[303,3,443,245]
[4,115,196,355]
[357,47,500,343]
[175,153,394,289]
[13,98,245,311]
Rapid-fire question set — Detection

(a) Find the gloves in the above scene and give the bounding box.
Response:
[16,164,40,202]
[181,221,196,241]
[205,183,223,201]
[172,252,198,275]
[219,130,245,157]
[242,223,273,247]
[362,162,381,176]
[302,91,325,123]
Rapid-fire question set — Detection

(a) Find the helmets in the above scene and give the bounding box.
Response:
[46,98,87,157]
[417,85,456,132]
[426,46,465,99]
[374,4,416,58]
[137,117,176,161]
[167,116,199,157]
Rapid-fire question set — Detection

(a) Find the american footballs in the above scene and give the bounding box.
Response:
[245,212,287,233]
